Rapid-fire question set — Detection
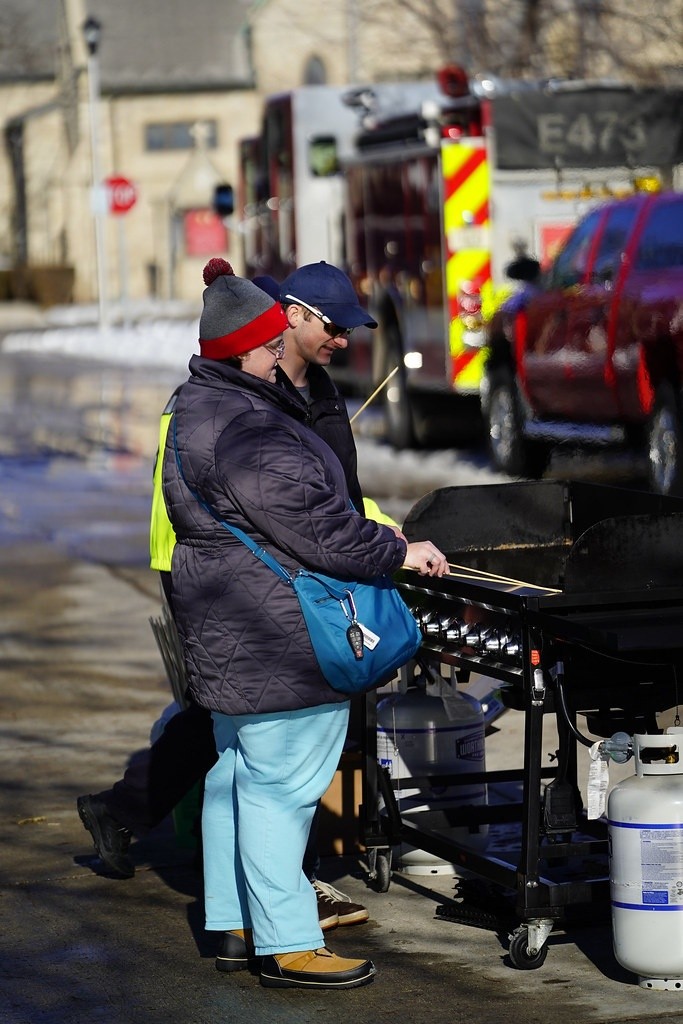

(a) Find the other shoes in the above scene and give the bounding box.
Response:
[214,927,259,975]
[259,946,378,989]
[77,789,138,879]
[320,880,370,926]
[306,878,343,932]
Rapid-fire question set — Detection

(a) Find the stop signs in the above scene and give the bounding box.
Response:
[105,177,137,215]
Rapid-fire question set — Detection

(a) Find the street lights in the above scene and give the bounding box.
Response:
[78,11,112,337]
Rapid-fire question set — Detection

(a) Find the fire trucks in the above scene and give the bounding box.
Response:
[234,63,682,453]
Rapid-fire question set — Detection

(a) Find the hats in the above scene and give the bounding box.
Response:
[276,260,380,331]
[198,258,290,362]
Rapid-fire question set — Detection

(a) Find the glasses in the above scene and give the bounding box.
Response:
[261,341,288,358]
[285,294,355,334]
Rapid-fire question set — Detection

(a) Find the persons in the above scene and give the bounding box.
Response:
[274,260,379,931]
[77,274,281,879]
[504,236,540,282]
[162,258,451,985]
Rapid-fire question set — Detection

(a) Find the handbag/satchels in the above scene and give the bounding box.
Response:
[281,563,428,693]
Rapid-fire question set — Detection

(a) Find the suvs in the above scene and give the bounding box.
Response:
[479,189,683,500]
